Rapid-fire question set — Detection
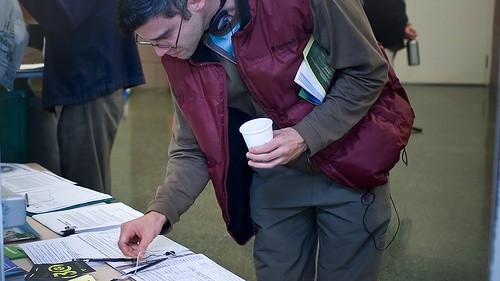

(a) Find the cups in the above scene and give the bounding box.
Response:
[239,117,273,149]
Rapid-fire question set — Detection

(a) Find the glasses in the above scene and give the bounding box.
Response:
[136,0,188,50]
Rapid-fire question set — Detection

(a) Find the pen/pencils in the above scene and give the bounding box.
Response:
[72,257,136,262]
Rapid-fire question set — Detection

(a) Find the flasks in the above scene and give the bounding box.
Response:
[407,40,420,65]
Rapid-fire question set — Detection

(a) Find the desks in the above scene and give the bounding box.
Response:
[1,163,251,281]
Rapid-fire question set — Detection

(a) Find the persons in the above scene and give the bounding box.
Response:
[116,1,417,281]
[361,1,418,69]
[0,0,28,99]
[19,1,150,194]
